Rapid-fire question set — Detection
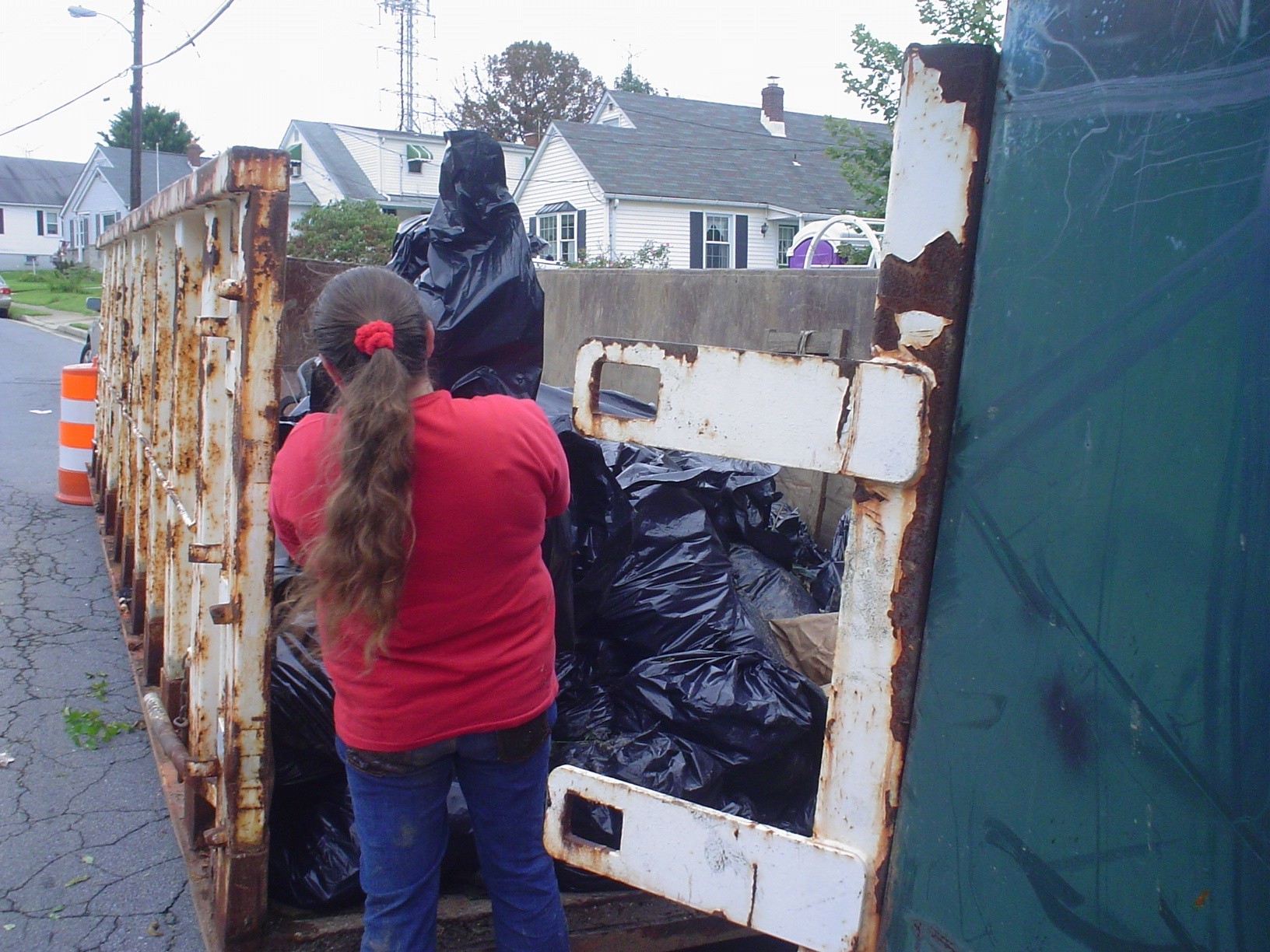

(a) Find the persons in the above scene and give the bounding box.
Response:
[269,268,572,952]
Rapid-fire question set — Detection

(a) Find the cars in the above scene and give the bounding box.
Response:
[0,276,12,317]
[79,297,101,364]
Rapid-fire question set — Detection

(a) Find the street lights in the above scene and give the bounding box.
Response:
[67,0,145,211]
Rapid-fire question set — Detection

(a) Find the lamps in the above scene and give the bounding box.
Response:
[761,223,769,238]
